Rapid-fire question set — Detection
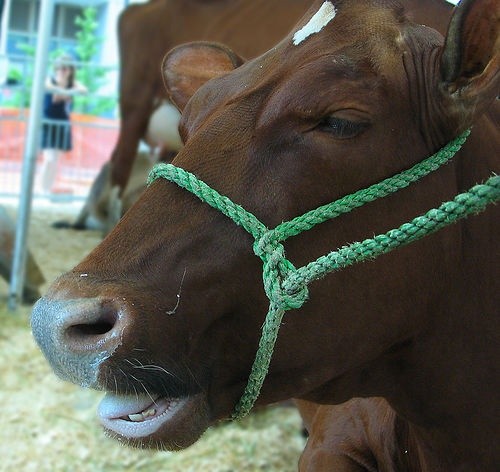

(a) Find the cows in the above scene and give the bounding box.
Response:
[30,1,499,472]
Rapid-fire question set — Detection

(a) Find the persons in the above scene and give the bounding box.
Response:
[42,53,90,199]
